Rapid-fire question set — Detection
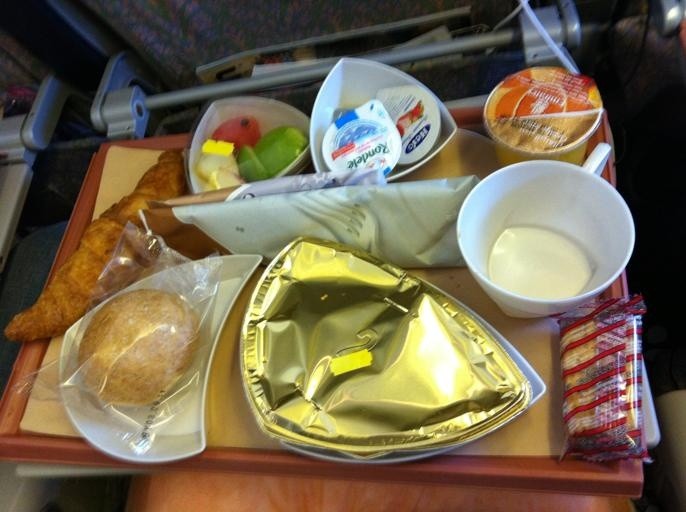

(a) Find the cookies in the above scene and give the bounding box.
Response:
[561,320,642,448]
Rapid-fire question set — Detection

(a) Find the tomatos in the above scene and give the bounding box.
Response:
[213,116,260,150]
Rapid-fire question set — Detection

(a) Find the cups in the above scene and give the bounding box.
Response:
[455,141,637,321]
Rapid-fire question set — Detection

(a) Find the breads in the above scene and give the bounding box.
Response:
[3,150,188,342]
[78,289,199,406]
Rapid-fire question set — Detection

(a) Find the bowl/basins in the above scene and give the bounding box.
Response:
[308,56,457,186]
[187,96,310,194]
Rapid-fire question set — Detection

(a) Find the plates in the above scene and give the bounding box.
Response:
[59,252,263,463]
[238,234,547,464]
